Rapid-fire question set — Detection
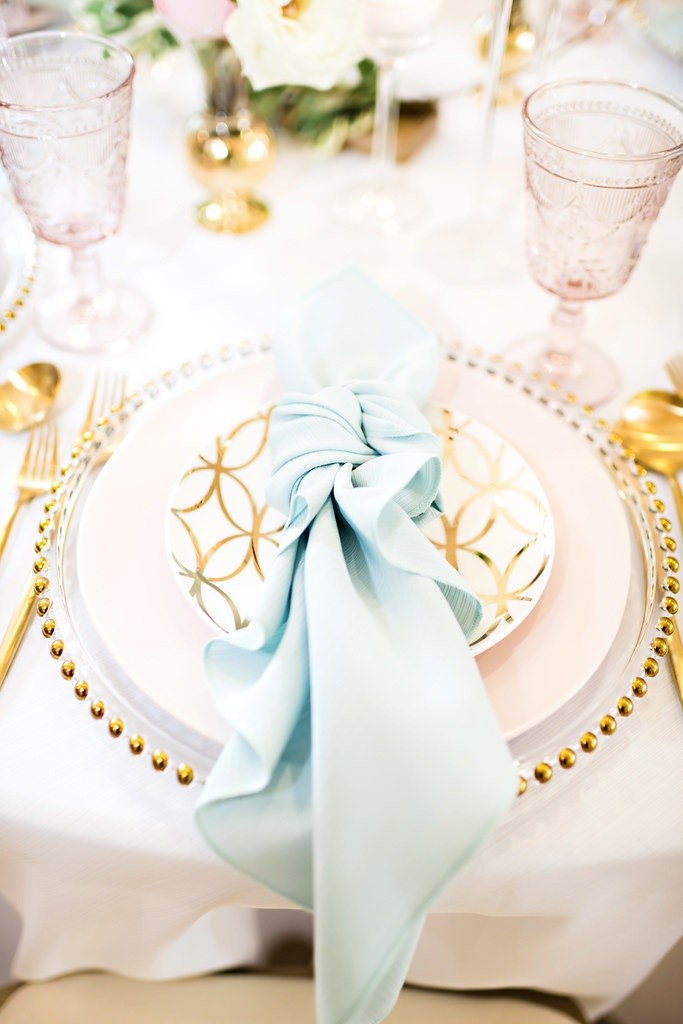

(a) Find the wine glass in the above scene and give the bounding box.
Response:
[510,79,683,414]
[0,31,151,351]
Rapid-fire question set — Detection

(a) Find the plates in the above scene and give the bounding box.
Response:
[35,332,680,804]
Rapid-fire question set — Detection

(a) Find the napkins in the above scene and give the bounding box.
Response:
[187,303,520,1024]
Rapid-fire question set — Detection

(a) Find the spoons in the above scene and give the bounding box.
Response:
[615,391,683,534]
[0,361,62,435]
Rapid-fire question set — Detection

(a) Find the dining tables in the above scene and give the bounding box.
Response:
[0,0,682,1024]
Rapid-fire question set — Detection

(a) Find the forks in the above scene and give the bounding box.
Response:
[0,377,129,690]
